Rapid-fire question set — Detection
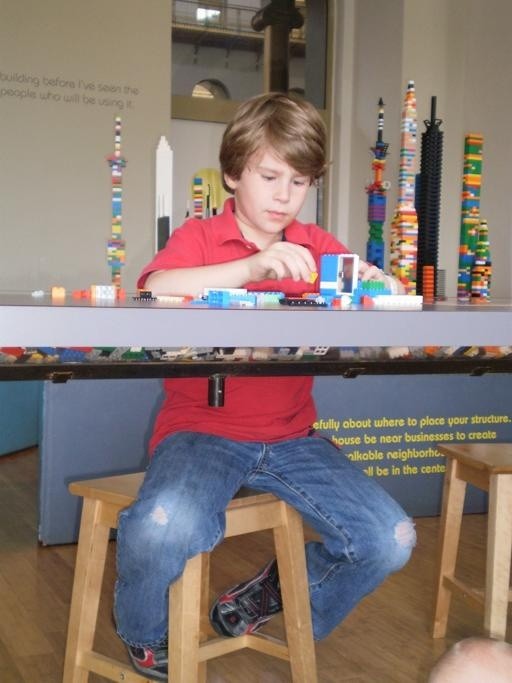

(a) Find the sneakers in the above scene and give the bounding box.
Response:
[211,560,283,640]
[110,612,168,678]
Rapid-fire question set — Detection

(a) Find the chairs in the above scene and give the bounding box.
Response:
[62,468,319,682]
[425,442,511,641]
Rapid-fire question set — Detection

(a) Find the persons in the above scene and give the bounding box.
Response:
[112,92,416,683]
